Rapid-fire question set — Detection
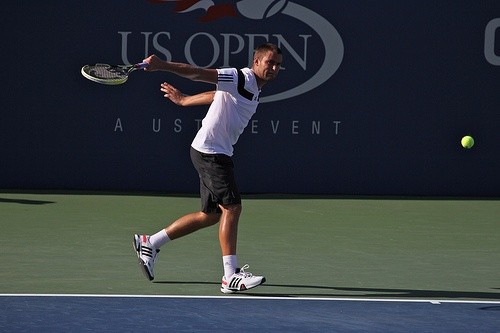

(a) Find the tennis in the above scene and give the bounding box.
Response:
[461,136,473,148]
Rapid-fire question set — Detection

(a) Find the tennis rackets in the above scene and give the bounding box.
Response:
[80,58,150,86]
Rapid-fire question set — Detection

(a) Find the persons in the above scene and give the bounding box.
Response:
[132,42,284,294]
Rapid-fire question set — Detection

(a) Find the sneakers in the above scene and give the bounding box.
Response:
[132,233,161,281]
[221,268,266,294]
